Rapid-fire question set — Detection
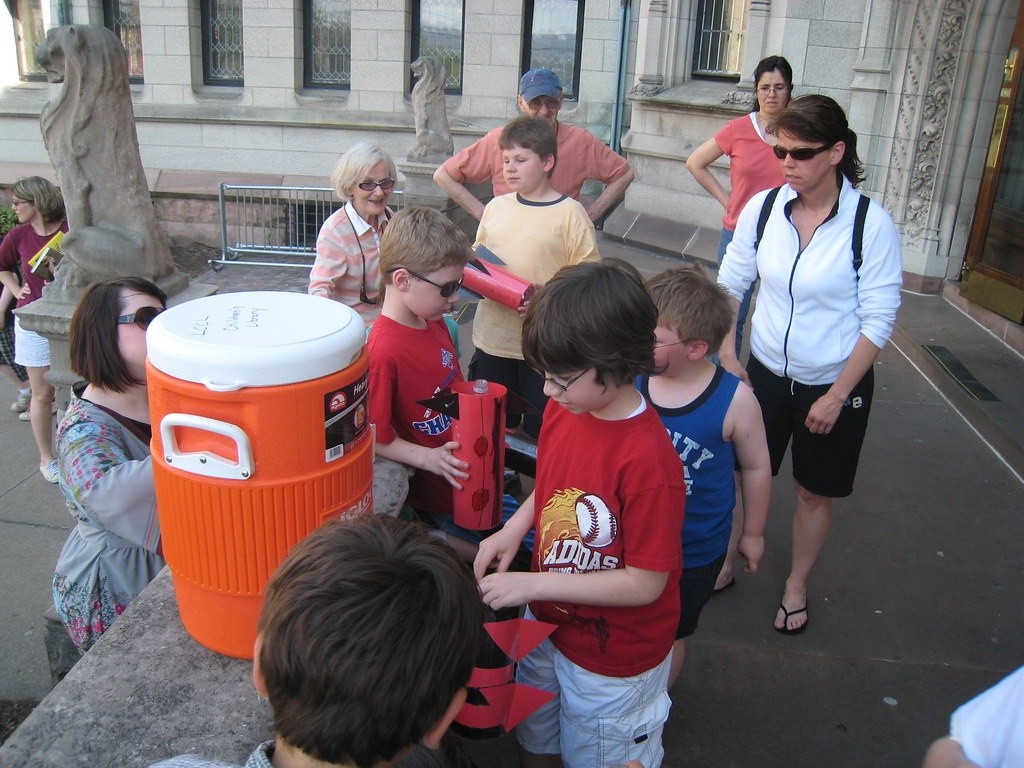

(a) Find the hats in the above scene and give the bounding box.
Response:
[519,69,561,104]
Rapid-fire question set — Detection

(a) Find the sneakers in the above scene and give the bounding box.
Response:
[10,392,32,412]
[39,455,60,484]
[18,398,58,420]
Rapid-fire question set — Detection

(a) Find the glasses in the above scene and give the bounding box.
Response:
[354,178,396,191]
[773,143,834,161]
[385,265,464,298]
[531,365,594,393]
[115,305,165,331]
[12,201,31,206]
[754,87,789,95]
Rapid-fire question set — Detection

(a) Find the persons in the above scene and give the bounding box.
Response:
[717,94,903,635]
[52,276,167,660]
[361,205,534,562]
[634,269,773,689]
[466,116,599,495]
[921,665,1024,768]
[0,177,68,483]
[473,262,686,768]
[686,56,794,365]
[137,513,483,768]
[308,144,396,326]
[434,68,635,222]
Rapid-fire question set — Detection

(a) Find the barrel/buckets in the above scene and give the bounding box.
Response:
[146,291,373,657]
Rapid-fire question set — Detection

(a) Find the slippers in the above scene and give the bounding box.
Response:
[709,575,736,593]
[770,596,808,635]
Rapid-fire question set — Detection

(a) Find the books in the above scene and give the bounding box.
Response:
[28,231,64,267]
[30,248,64,283]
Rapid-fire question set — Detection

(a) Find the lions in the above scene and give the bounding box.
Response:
[407,55,454,164]
[35,24,175,287]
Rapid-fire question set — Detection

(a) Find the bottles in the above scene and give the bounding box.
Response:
[470,379,488,395]
